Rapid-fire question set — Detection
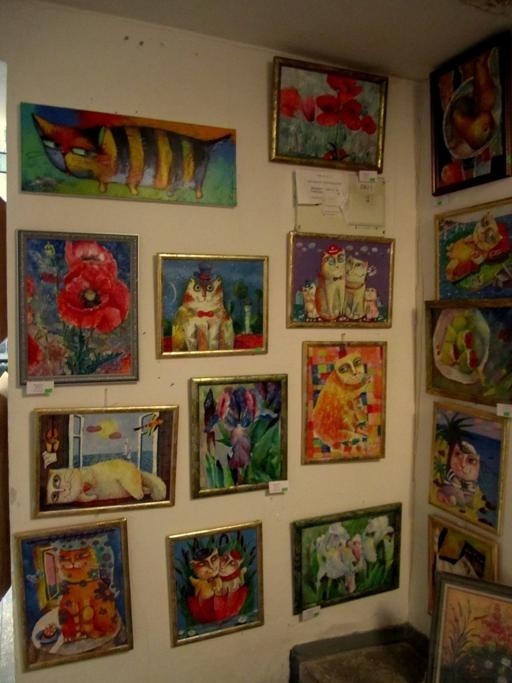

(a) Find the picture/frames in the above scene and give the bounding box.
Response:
[285,229,396,329]
[427,25,512,195]
[424,297,510,406]
[268,55,389,174]
[14,228,140,386]
[429,571,512,683]
[164,518,265,649]
[187,372,289,501]
[428,514,501,582]
[29,403,180,520]
[434,198,512,299]
[289,500,404,617]
[300,339,388,467]
[154,251,270,361]
[424,402,511,535]
[12,516,134,674]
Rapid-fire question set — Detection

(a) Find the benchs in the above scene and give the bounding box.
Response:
[288,623,430,682]
